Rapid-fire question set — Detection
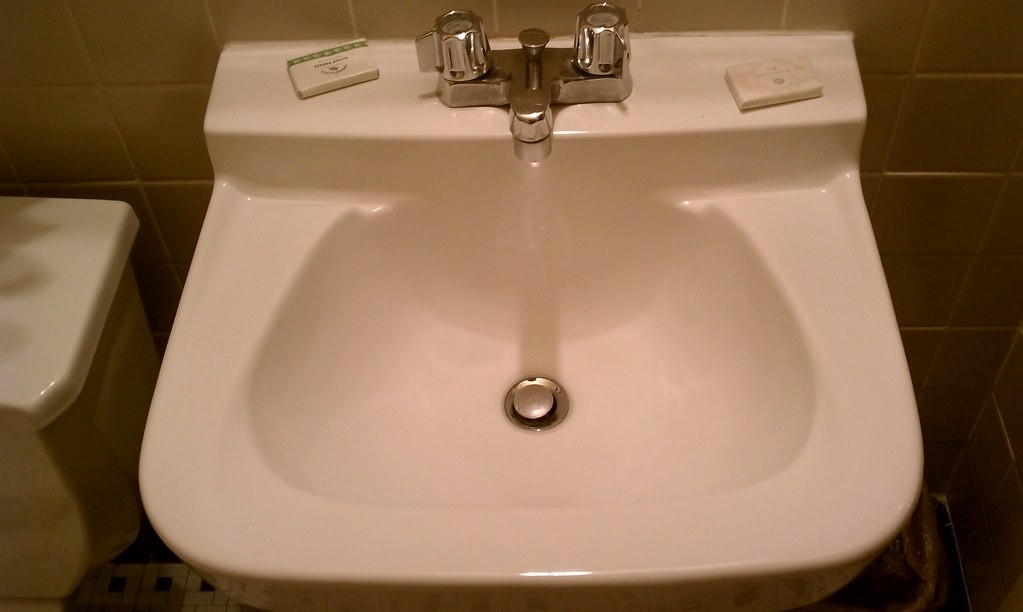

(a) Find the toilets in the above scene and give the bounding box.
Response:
[0,186,153,612]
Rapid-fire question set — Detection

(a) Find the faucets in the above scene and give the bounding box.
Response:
[501,22,564,171]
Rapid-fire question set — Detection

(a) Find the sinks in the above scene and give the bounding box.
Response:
[136,25,929,612]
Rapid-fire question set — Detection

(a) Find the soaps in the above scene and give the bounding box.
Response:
[286,34,381,103]
[721,53,827,114]
